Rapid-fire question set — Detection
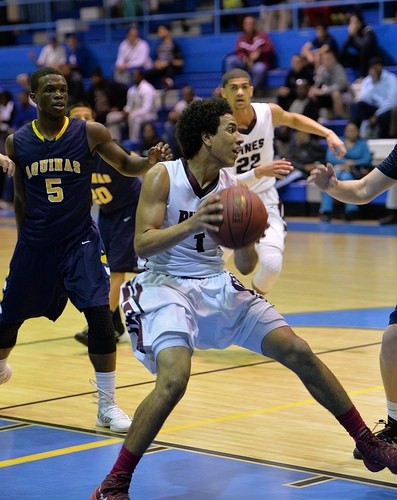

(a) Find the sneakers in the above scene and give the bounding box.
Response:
[74,318,125,347]
[90,471,133,500]
[96,405,133,435]
[0,362,14,386]
[353,416,397,474]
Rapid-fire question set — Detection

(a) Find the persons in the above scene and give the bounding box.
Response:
[0,88,17,155]
[0,154,16,178]
[377,184,397,226]
[14,33,67,97]
[65,105,149,348]
[6,91,37,137]
[59,21,223,157]
[273,129,325,221]
[318,120,371,223]
[223,15,276,98]
[88,97,396,500]
[275,10,396,159]
[304,139,397,461]
[0,66,174,434]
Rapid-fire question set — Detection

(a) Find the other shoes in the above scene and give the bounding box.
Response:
[317,208,331,223]
[346,209,359,221]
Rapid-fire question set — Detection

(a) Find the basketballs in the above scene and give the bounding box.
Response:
[206,187,267,249]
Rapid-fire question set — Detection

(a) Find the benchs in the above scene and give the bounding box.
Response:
[0,23,397,220]
